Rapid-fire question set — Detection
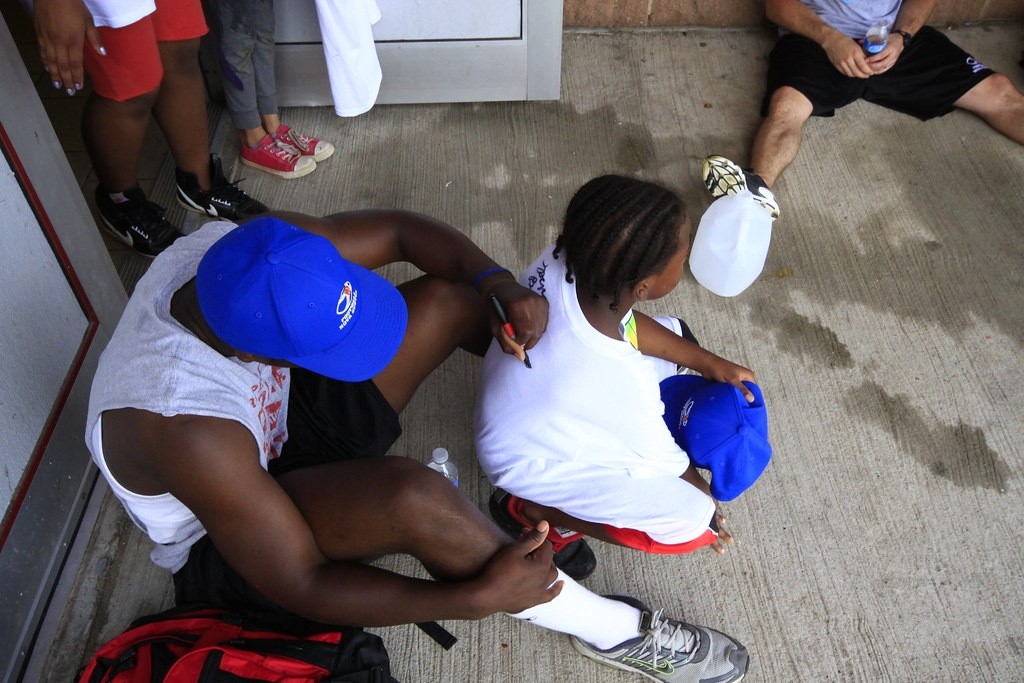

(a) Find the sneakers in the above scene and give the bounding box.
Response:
[489,488,596,580]
[239,132,316,179]
[175,153,269,223]
[274,124,335,162]
[700,155,779,224]
[572,594,749,683]
[95,183,187,260]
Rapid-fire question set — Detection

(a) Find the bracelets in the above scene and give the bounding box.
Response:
[473,267,511,285]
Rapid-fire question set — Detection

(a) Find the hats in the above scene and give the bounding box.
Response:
[196,216,409,382]
[660,374,772,503]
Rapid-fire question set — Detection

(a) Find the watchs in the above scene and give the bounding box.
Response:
[894,29,912,48]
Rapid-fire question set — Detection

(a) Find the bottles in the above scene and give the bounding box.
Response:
[427,447,458,490]
[864,20,889,58]
[688,189,773,296]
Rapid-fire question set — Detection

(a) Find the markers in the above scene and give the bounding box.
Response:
[491,296,531,368]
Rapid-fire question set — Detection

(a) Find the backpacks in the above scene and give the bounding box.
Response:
[80,603,397,683]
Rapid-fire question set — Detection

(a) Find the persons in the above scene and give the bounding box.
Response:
[702,0,1024,221]
[85,209,749,683]
[202,1,335,179]
[26,0,270,257]
[472,175,758,582]
